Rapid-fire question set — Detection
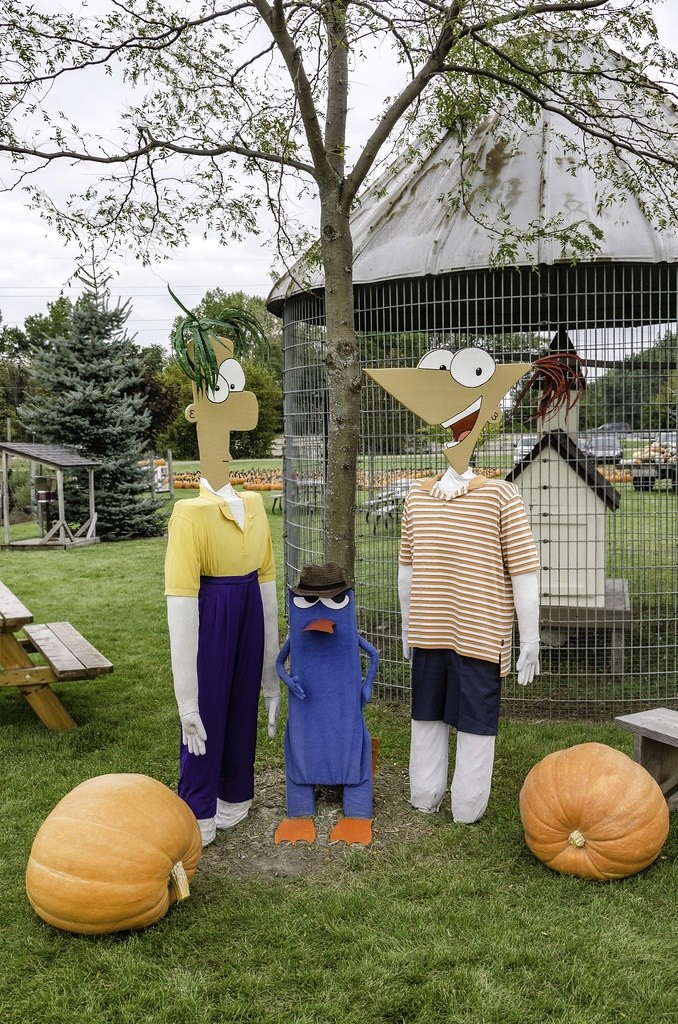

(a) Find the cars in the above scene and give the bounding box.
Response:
[578,421,678,492]
[513,436,538,466]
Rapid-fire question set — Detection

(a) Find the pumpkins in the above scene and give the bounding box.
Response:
[25,773,201,933]
[473,467,504,478]
[137,459,202,489]
[519,742,669,879]
[597,442,678,483]
[229,467,283,490]
[356,468,434,486]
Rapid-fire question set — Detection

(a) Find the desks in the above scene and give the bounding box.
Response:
[0,582,78,732]
[391,478,414,488]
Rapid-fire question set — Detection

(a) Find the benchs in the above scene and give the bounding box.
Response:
[615,707,678,814]
[363,490,407,536]
[24,621,114,679]
[272,494,283,514]
